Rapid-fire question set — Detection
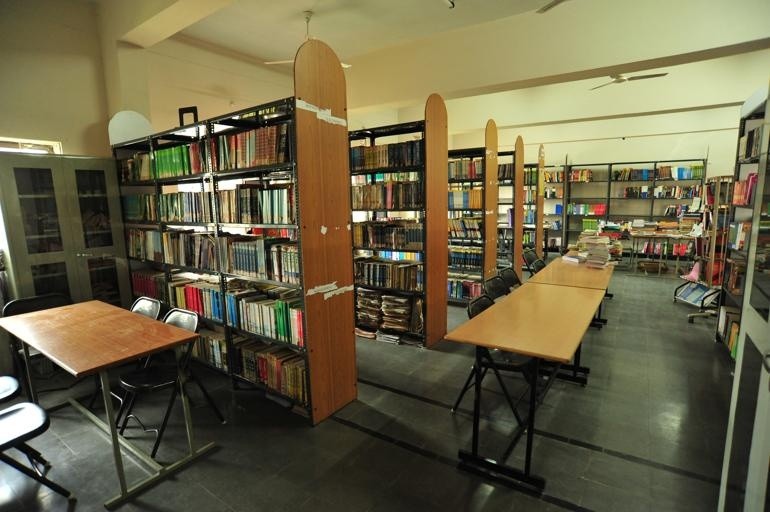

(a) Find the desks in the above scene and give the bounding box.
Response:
[444,283,606,490]
[2,300,218,510]
[630,228,697,280]
[525,254,615,375]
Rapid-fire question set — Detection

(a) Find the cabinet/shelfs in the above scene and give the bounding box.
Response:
[707,175,733,288]
[459,144,545,273]
[496,154,567,253]
[2,152,126,347]
[243,93,448,348]
[715,92,770,355]
[562,157,705,269]
[365,119,497,309]
[442,136,524,292]
[112,99,358,427]
[718,97,770,511]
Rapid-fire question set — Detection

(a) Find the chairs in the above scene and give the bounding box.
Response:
[0,376,19,404]
[117,306,228,459]
[497,267,523,291]
[452,295,552,434]
[0,402,74,498]
[2,297,99,406]
[87,296,164,424]
[470,276,550,382]
[521,249,546,277]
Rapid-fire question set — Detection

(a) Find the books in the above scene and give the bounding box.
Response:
[566,202,608,216]
[521,168,537,267]
[543,170,563,230]
[708,124,765,360]
[613,164,704,198]
[447,277,483,302]
[497,162,516,179]
[603,199,702,256]
[351,137,425,211]
[352,219,425,337]
[447,218,483,272]
[117,122,302,286]
[567,168,594,183]
[446,157,484,211]
[582,219,599,231]
[130,266,310,404]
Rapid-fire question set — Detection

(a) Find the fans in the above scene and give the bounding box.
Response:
[264,10,353,71]
